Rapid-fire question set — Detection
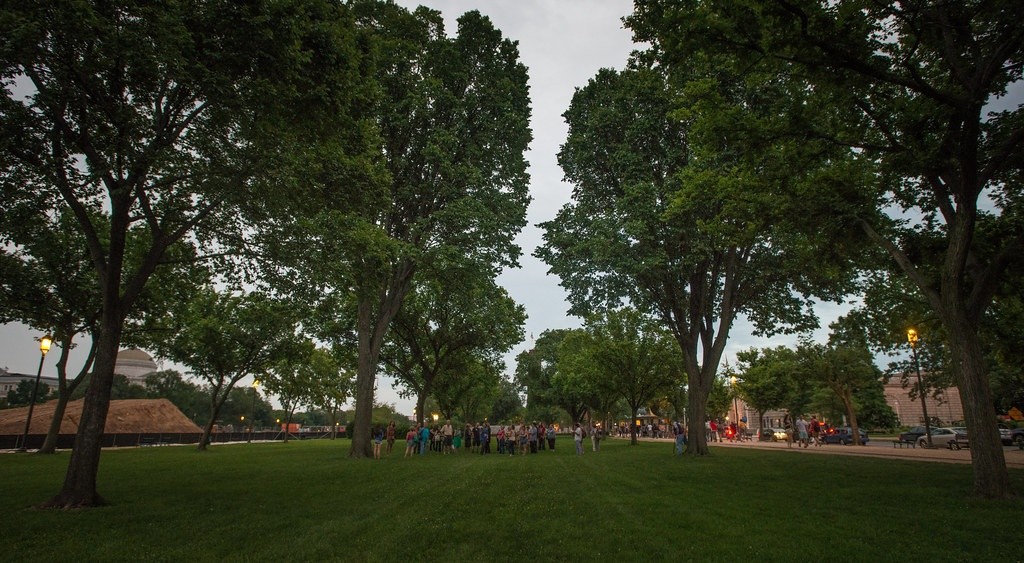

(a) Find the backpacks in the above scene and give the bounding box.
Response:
[813,419,820,432]
[582,428,586,438]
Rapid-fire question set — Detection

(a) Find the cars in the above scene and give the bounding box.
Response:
[763,427,789,442]
[917,426,970,451]
[898,425,939,443]
[996,422,1024,446]
[822,427,869,445]
[791,422,831,443]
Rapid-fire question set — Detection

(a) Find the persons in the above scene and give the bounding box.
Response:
[373,424,384,459]
[590,421,599,452]
[730,421,737,442]
[795,414,810,448]
[670,419,684,456]
[809,415,822,446]
[619,421,688,439]
[503,425,516,456]
[705,419,724,442]
[519,425,528,457]
[546,424,556,452]
[738,419,747,439]
[571,423,582,456]
[439,420,453,455]
[529,422,537,454]
[387,420,396,454]
[402,423,461,459]
[464,422,491,456]
[495,421,545,454]
[783,414,793,448]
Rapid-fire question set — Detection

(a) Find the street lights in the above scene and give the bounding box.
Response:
[907,328,937,451]
[731,375,739,430]
[15,332,54,453]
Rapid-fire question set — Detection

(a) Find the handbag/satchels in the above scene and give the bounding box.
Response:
[413,433,419,442]
[785,425,793,435]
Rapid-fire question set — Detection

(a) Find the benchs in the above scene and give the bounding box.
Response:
[948,434,969,450]
[135,437,158,447]
[156,436,173,446]
[892,433,921,449]
